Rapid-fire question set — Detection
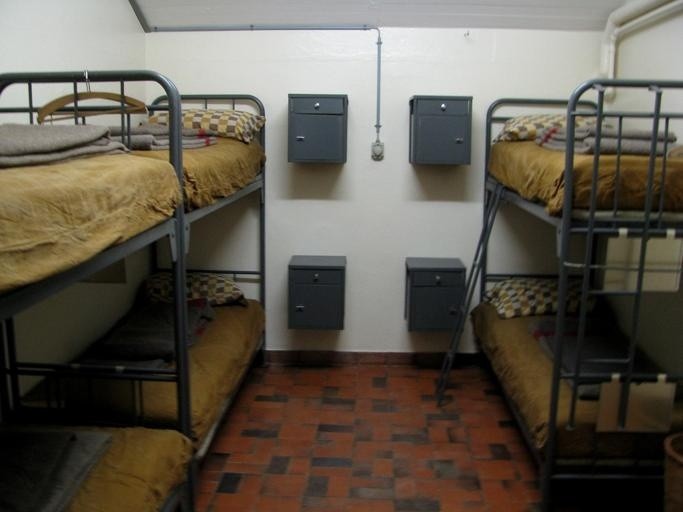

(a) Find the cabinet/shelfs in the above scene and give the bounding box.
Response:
[405,257,466,332]
[409,95,471,166]
[288,94,347,165]
[287,255,345,331]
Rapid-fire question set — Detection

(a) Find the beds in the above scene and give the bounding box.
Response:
[0,69,264,507]
[469,79,682,510]
[0,71,194,512]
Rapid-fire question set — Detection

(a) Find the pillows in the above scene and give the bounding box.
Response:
[494,116,614,141]
[483,279,593,319]
[156,271,245,309]
[142,109,263,143]
